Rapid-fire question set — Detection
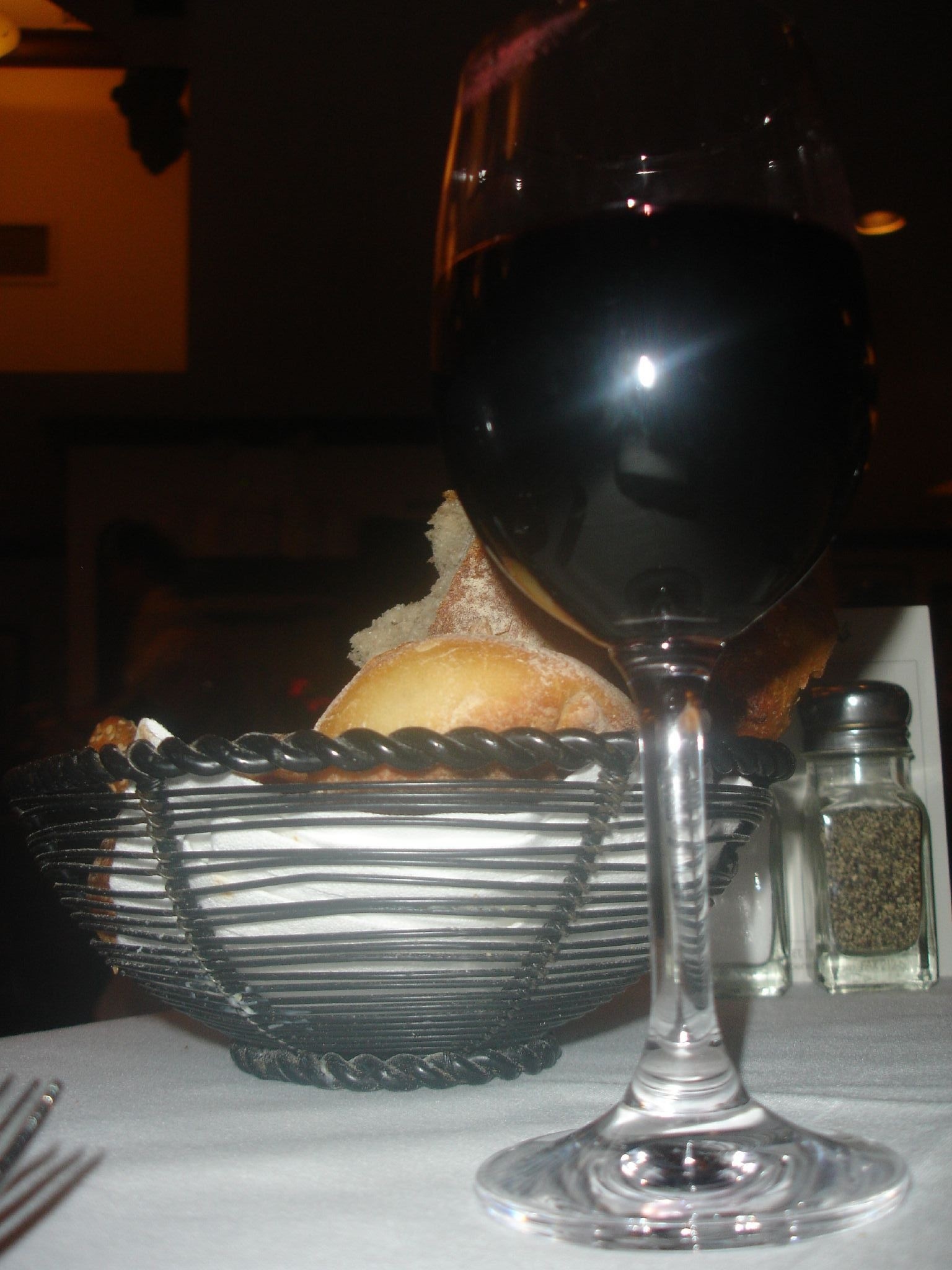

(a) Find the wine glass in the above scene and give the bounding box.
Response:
[436,59,912,1253]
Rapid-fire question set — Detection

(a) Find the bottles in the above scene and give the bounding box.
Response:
[796,682,942,996]
[699,744,792,996]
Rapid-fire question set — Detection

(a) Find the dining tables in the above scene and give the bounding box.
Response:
[0,985,952,1270]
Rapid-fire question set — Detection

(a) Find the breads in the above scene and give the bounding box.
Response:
[83,489,836,788]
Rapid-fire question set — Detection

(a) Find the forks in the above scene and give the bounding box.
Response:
[0,1073,63,1175]
